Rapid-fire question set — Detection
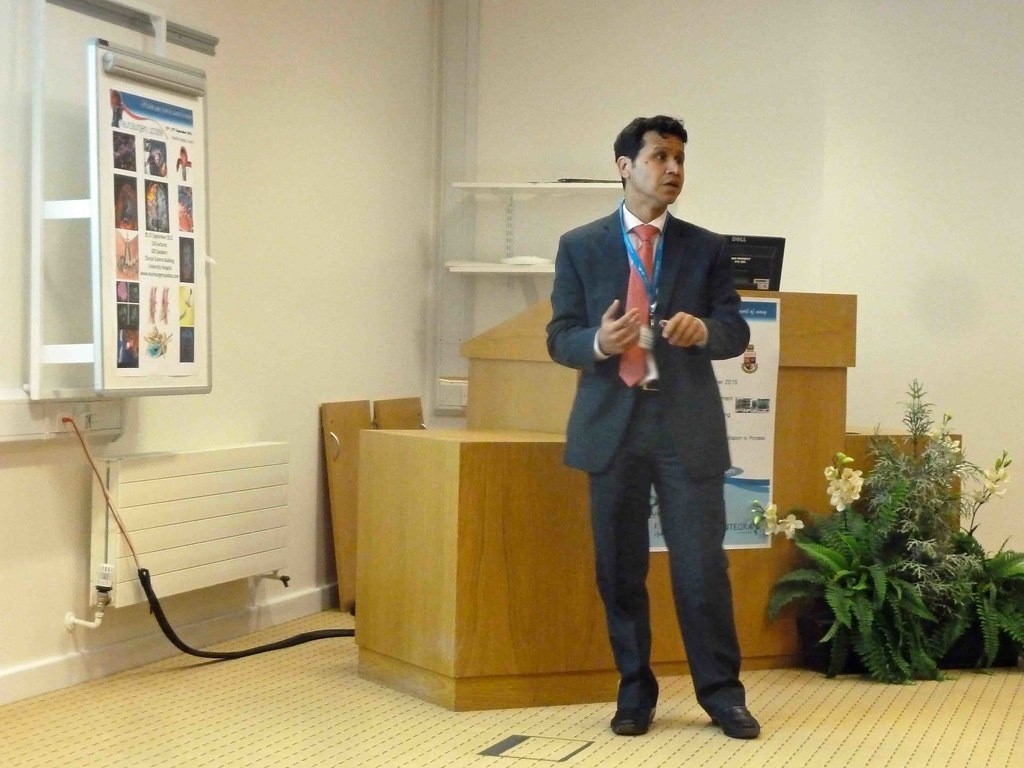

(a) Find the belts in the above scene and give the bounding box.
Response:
[640,380,660,391]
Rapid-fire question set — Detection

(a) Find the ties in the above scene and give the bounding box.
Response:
[619,224,660,387]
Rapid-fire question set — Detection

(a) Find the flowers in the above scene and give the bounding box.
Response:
[751,381,1024,684]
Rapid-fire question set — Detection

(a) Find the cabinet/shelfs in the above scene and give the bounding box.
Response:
[446,181,627,275]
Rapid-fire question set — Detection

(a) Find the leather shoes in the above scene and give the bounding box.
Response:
[712,704,760,739]
[611,705,656,735]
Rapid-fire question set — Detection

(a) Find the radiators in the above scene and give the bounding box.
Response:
[57,444,297,630]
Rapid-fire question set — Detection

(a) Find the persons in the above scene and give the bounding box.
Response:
[546,115,760,739]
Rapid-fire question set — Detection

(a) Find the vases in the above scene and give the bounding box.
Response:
[960,619,1019,670]
[800,604,865,671]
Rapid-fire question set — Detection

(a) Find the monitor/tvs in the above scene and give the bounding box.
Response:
[723,235,786,291]
